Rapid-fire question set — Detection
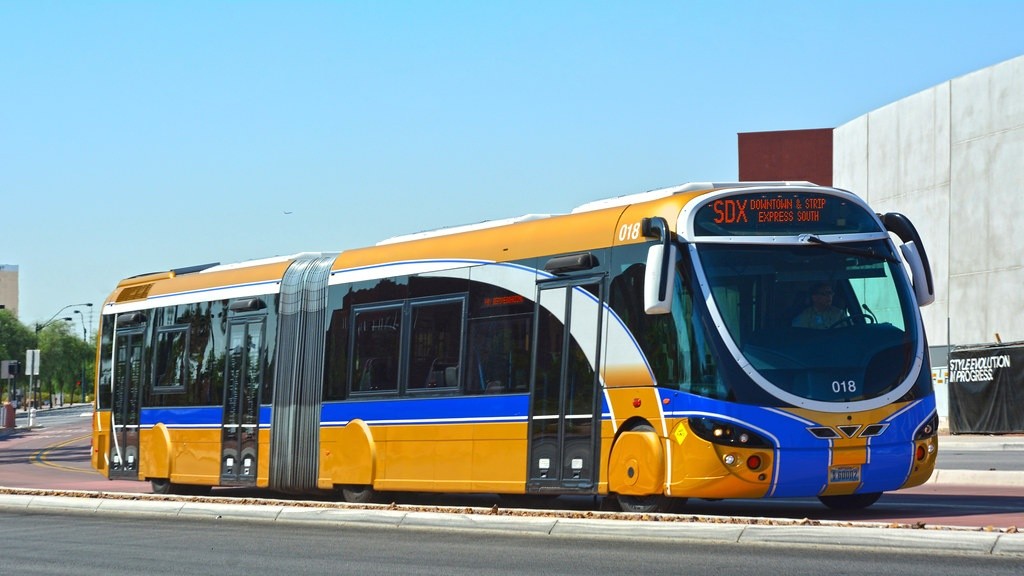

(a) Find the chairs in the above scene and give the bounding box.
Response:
[360,358,394,390]
[427,356,449,389]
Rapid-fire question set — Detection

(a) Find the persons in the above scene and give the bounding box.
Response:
[792,281,853,329]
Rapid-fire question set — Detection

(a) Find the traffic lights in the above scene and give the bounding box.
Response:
[8,363,20,376]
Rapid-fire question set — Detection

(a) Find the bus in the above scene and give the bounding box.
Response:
[89,177,943,514]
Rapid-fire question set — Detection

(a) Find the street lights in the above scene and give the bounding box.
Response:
[33,302,93,412]
